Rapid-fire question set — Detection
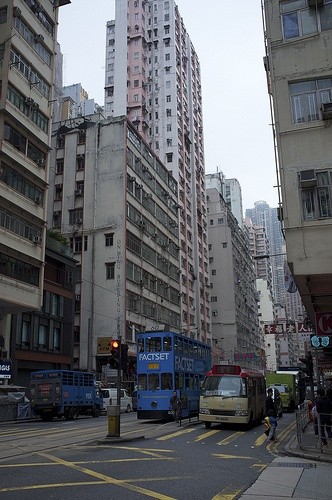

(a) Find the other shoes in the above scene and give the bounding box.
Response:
[265,439,270,443]
[274,440,279,442]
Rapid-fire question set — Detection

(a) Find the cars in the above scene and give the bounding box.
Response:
[306,386,317,399]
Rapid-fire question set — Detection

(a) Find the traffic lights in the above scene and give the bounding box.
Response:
[299,356,312,384]
[109,339,120,368]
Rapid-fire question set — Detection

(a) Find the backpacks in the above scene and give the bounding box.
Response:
[264,417,271,431]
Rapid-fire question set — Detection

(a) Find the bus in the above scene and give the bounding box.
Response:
[199,365,266,431]
[136,330,211,419]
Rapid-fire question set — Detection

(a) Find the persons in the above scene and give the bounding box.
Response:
[264,388,277,443]
[170,391,181,423]
[316,388,332,448]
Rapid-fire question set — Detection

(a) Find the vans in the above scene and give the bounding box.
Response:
[96,389,134,413]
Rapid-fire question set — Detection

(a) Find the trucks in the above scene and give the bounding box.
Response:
[266,370,301,418]
[29,370,103,421]
